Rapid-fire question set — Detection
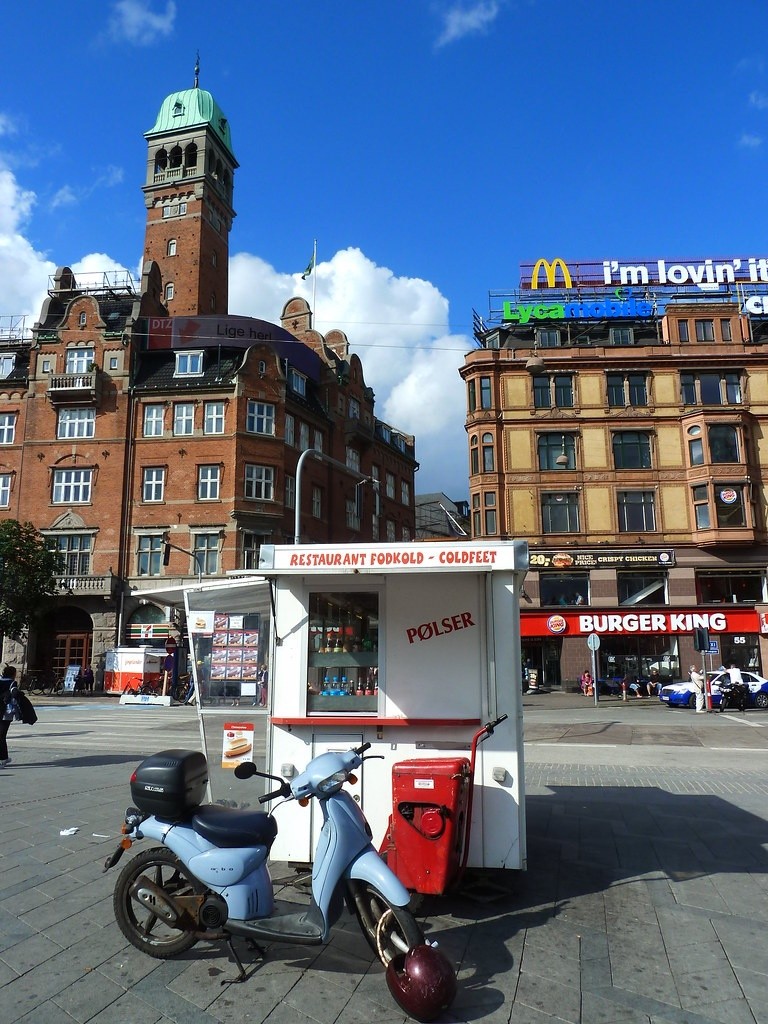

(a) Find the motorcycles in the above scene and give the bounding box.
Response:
[715,681,750,714]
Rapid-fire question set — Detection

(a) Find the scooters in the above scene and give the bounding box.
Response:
[102,742,439,987]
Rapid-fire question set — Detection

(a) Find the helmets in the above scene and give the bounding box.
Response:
[386,945,458,1021]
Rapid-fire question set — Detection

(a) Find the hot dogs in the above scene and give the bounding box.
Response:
[224,738,252,757]
[211,619,257,678]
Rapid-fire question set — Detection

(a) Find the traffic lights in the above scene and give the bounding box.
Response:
[693,628,703,651]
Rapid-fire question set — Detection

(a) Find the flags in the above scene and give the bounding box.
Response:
[301,254,314,281]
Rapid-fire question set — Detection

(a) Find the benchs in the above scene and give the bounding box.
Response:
[608,677,650,695]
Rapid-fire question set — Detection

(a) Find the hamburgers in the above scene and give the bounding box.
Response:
[552,553,573,567]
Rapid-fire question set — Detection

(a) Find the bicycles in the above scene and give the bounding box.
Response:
[18,666,65,698]
[127,671,192,703]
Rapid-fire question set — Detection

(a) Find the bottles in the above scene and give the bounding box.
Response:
[321,676,378,698]
[310,625,378,654]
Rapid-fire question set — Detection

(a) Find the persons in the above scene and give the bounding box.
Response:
[231,698,240,706]
[689,664,707,713]
[257,665,268,707]
[629,670,662,699]
[580,670,593,697]
[185,660,205,706]
[558,591,585,606]
[84,664,94,691]
[0,666,24,769]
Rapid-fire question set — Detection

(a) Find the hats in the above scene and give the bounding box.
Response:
[261,665,267,669]
[196,660,205,666]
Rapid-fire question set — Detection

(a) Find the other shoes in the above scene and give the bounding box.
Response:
[184,700,188,706]
[231,704,235,706]
[0,758,13,770]
[259,703,267,707]
[187,701,195,706]
[236,703,240,707]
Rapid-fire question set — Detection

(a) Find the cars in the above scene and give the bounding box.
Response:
[658,670,768,710]
[590,668,652,695]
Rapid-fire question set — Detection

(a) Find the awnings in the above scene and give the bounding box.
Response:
[128,576,277,804]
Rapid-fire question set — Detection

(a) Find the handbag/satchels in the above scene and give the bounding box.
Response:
[2,707,15,721]
